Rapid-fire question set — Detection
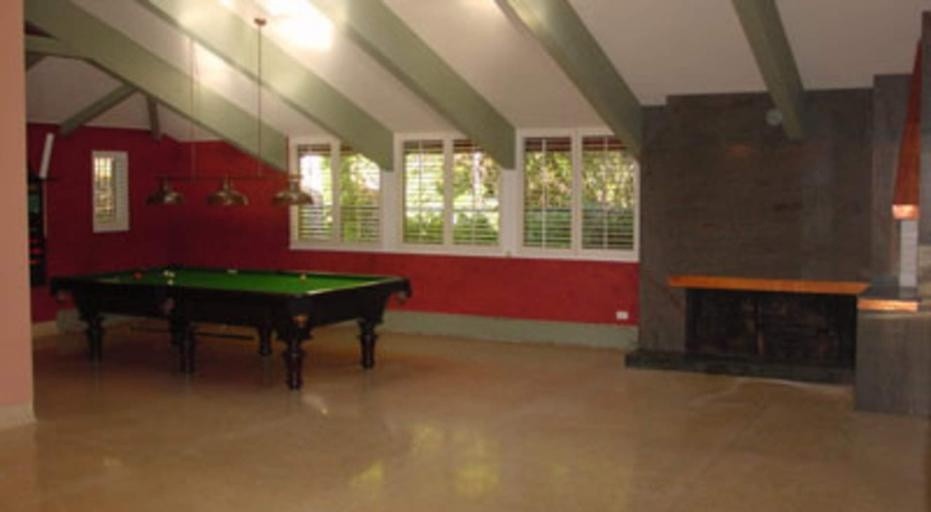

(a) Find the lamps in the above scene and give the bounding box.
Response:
[204,171,248,206]
[144,173,183,204]
[271,175,313,205]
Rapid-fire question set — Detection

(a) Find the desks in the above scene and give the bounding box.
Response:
[664,273,872,379]
[51,266,406,389]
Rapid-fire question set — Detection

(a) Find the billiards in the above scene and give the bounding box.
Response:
[163,270,175,286]
[300,274,307,280]
[132,273,141,279]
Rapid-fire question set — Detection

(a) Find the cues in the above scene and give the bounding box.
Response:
[130,326,255,338]
